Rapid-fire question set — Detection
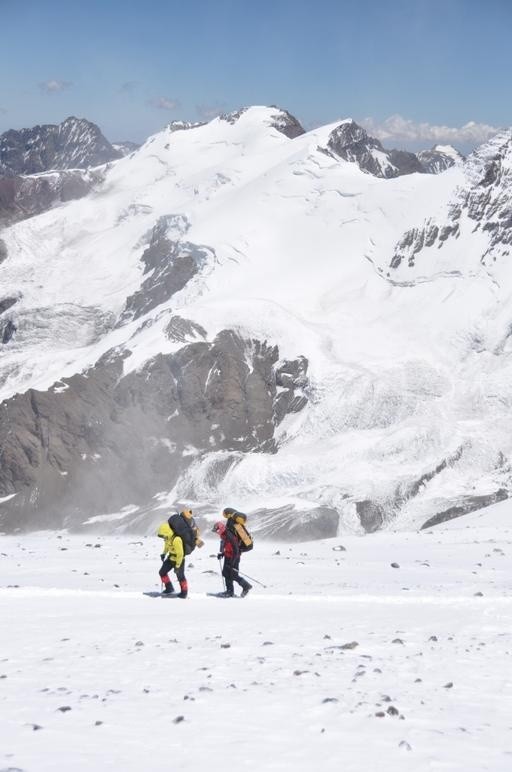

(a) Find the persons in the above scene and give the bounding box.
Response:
[158,522,188,599]
[211,522,252,598]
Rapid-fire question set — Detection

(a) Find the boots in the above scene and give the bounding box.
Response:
[163,582,174,593]
[177,592,187,598]
[219,585,234,596]
[239,579,252,597]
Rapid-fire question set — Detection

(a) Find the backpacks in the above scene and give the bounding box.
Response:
[225,518,253,551]
[168,514,195,557]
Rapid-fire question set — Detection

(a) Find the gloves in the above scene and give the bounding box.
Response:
[161,554,165,560]
[218,553,224,559]
[230,559,236,567]
[174,567,180,573]
